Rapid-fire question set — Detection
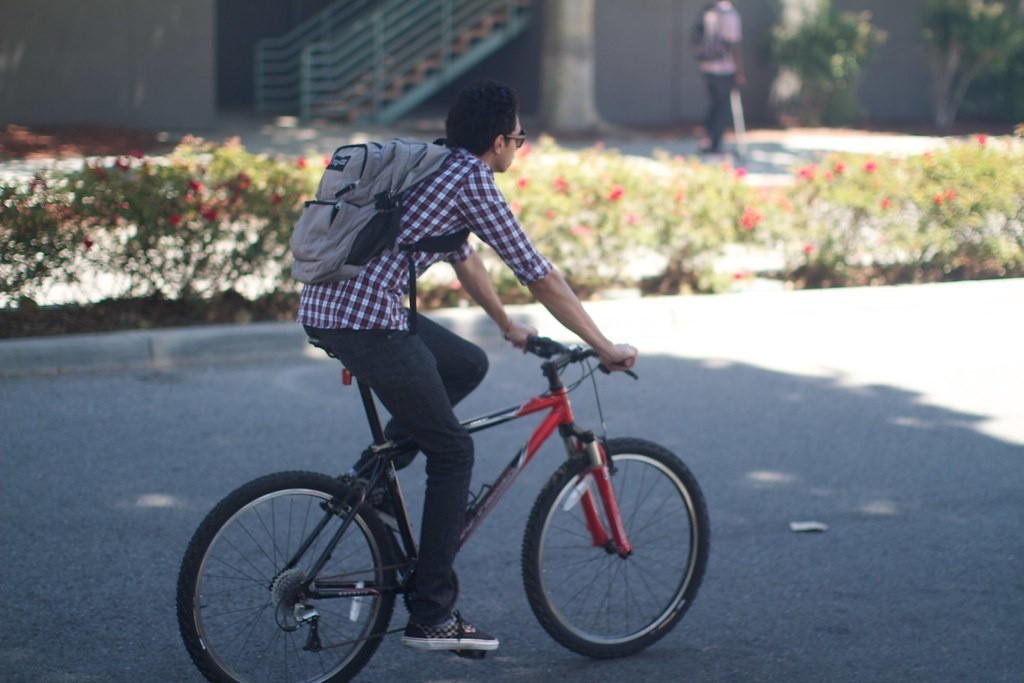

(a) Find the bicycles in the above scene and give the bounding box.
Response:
[176,335,710,682]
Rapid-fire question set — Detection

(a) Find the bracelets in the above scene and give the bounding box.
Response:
[503,318,513,335]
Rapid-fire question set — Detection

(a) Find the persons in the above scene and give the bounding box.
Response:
[695,0,744,156]
[300,78,637,650]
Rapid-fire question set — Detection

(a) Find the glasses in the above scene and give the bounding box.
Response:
[507,128,527,148]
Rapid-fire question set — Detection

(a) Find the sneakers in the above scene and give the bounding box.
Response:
[335,467,413,534]
[400,608,500,652]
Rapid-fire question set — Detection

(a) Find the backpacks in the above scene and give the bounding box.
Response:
[689,0,738,62]
[291,138,472,285]
